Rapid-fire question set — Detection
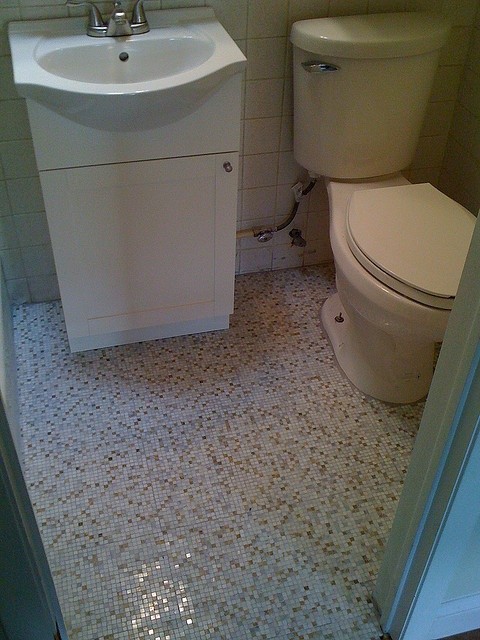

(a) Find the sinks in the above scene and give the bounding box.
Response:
[8,7,247,136]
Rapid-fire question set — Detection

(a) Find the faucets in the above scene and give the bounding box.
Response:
[62,0,163,35]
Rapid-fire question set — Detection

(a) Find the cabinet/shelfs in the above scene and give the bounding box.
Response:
[38,152,241,353]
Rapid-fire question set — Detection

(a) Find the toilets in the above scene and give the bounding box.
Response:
[290,11,476,405]
